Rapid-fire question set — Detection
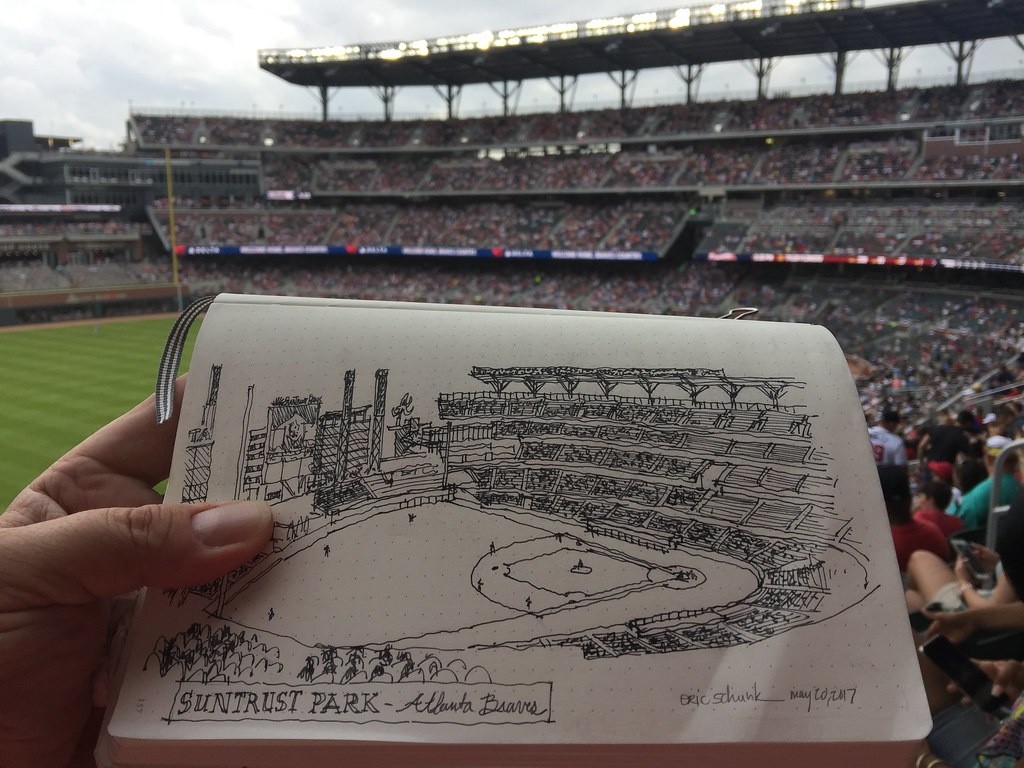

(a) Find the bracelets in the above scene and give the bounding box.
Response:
[959,583,978,598]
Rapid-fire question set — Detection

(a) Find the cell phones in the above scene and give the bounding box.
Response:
[950,541,990,581]
[921,634,1011,714]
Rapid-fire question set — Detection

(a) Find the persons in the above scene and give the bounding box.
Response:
[853,365,1024,768]
[0,370,273,768]
[1,79,1024,365]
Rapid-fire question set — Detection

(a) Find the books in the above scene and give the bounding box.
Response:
[93,294,934,767]
